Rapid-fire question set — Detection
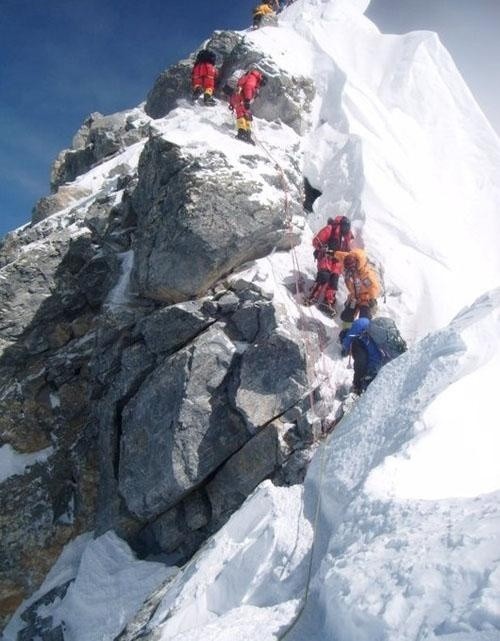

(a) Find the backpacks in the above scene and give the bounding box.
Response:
[362,317,409,364]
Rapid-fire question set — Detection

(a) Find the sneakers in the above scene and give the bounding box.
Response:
[190,85,218,106]
[235,129,257,147]
[304,295,336,318]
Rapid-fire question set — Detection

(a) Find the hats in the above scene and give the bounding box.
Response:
[359,304,373,321]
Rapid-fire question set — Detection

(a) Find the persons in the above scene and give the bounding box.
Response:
[335,319,383,399]
[301,214,355,319]
[252,0,293,29]
[323,247,383,329]
[227,68,269,147]
[191,48,219,107]
